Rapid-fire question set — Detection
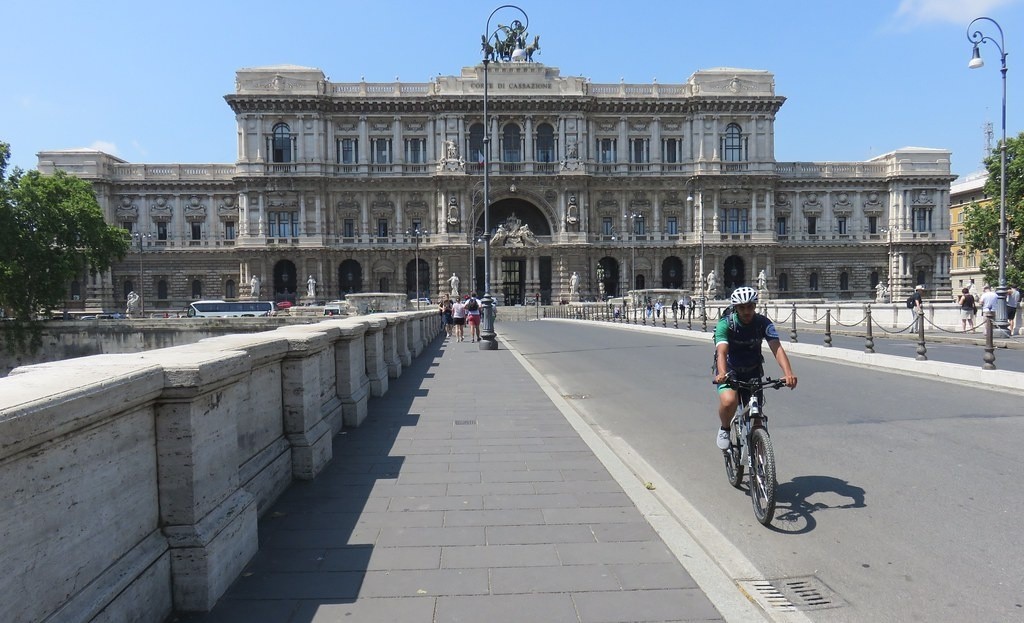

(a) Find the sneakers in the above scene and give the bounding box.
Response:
[717,426,730,449]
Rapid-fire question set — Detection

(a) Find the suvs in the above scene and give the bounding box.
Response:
[276,301,292,310]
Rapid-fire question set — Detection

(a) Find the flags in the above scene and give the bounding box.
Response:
[479,149,484,166]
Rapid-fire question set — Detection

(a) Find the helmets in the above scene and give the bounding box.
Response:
[730,286,759,305]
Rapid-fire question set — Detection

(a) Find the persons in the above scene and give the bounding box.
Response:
[758,269,767,289]
[647,301,652,318]
[959,288,976,333]
[875,280,885,298]
[979,286,997,336]
[307,274,316,296]
[438,291,498,343]
[672,300,678,314]
[909,285,924,334]
[707,270,717,292]
[679,298,686,319]
[251,275,260,296]
[1006,284,1020,336]
[447,273,460,296]
[656,301,662,318]
[569,271,580,293]
[711,286,798,451]
[689,298,696,319]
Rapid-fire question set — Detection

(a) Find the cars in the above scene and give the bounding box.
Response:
[418,298,431,305]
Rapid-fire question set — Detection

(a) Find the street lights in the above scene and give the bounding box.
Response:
[881,225,900,302]
[469,179,491,297]
[622,210,636,309]
[478,4,530,351]
[611,225,625,307]
[404,227,429,310]
[685,178,707,320]
[130,231,151,320]
[967,17,1011,340]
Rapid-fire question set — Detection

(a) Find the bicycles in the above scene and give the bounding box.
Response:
[711,374,787,524]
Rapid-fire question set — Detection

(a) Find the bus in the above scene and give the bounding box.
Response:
[183,300,277,318]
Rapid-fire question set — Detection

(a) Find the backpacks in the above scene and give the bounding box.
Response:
[468,299,478,311]
[907,297,914,308]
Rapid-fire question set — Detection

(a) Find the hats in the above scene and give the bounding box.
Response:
[916,285,924,290]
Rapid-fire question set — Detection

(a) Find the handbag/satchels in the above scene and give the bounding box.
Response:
[712,306,735,375]
[973,303,977,314]
